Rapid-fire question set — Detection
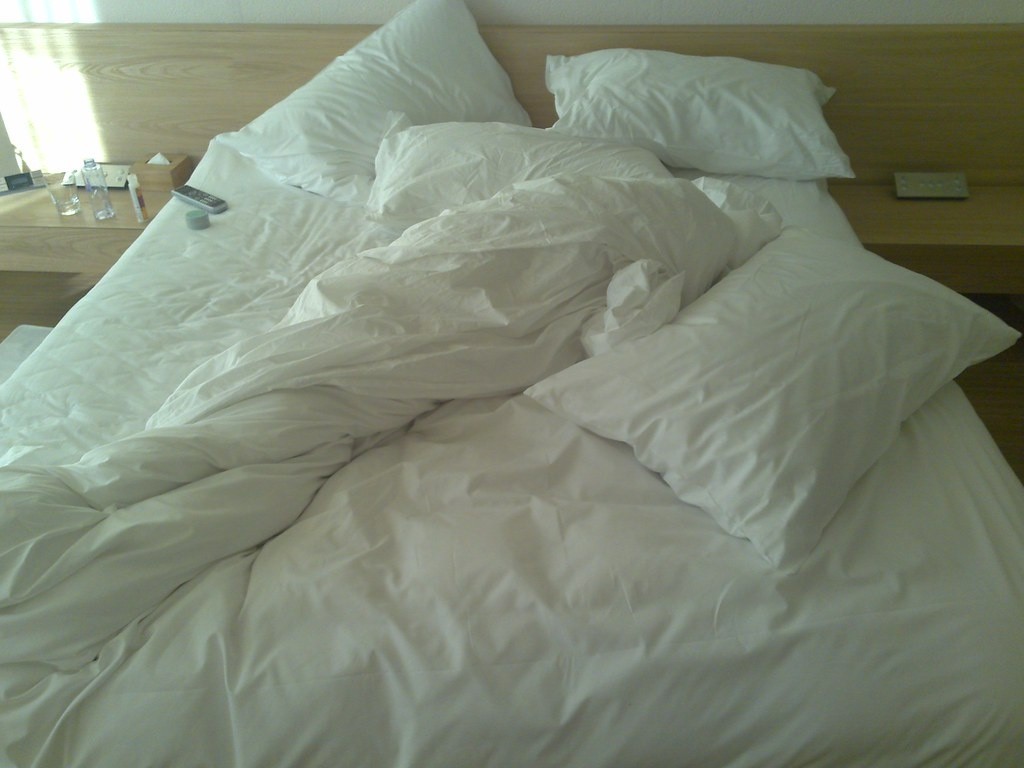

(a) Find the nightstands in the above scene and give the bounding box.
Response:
[0,168,195,274]
[830,184,1024,295]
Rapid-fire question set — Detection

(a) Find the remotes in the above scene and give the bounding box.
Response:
[171,184,228,214]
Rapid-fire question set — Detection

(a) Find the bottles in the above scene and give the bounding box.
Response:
[81,159,114,219]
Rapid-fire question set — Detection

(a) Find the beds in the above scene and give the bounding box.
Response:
[0,0,1024,768]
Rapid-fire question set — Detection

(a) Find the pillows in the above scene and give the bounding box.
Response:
[217,0,535,211]
[365,111,675,228]
[546,48,857,182]
[524,224,1022,569]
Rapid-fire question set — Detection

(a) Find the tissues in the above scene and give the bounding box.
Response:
[129,150,195,193]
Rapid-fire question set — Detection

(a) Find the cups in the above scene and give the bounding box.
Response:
[45,172,80,216]
